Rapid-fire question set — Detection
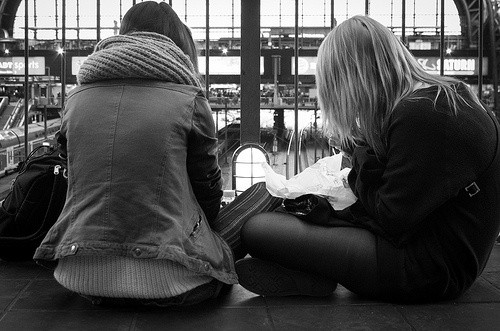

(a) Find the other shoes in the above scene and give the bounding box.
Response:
[235,258,338,296]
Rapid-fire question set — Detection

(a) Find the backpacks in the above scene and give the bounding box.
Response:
[0,145,68,262]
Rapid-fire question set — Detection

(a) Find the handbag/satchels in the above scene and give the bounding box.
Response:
[275,194,350,226]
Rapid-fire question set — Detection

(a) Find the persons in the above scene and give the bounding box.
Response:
[209,86,240,105]
[51,92,61,106]
[260,85,305,106]
[234,15,500,305]
[33,0,283,309]
[4,86,24,106]
